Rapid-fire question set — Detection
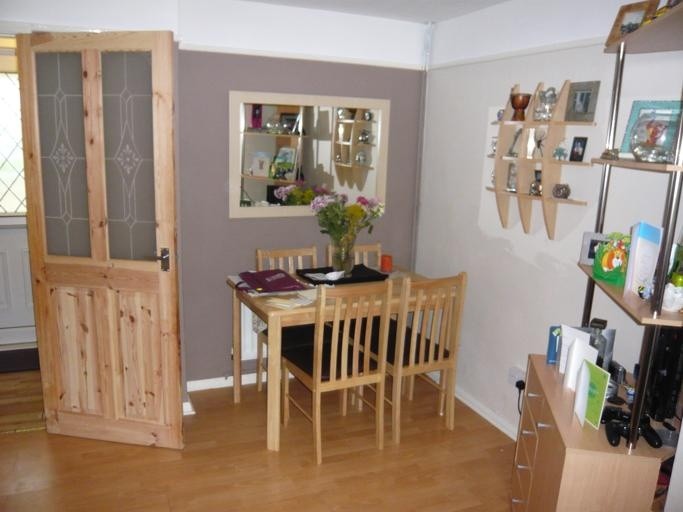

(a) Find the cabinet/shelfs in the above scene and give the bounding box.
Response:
[239,103,311,188]
[504,353,683,511]
[483,77,592,241]
[575,1,683,451]
[330,106,379,172]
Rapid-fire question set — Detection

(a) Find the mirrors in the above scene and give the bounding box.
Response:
[226,89,391,221]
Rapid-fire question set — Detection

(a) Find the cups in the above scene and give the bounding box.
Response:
[380,254,392,273]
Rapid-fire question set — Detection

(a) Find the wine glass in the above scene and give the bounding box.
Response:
[509,94,530,121]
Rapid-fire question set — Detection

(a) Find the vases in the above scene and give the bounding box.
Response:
[328,235,356,272]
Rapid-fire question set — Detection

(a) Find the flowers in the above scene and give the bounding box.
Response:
[309,190,388,252]
[271,176,336,207]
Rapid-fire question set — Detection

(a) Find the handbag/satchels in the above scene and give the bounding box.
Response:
[234,269,305,295]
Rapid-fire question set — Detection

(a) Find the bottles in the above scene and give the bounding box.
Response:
[334,109,371,164]
[587,317,606,367]
[626,387,634,399]
[265,120,287,134]
[630,116,675,162]
[533,88,556,121]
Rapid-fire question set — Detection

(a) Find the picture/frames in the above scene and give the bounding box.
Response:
[278,111,306,137]
[567,135,588,163]
[563,79,601,123]
[616,97,682,165]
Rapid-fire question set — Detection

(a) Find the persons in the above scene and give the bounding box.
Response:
[574,141,584,159]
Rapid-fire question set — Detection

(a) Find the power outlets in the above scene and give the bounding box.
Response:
[506,362,527,390]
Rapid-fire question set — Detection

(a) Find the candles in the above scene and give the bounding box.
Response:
[379,254,392,274]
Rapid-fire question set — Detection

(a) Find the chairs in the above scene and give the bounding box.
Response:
[254,245,346,420]
[278,278,395,467]
[341,268,467,443]
[324,240,411,410]
